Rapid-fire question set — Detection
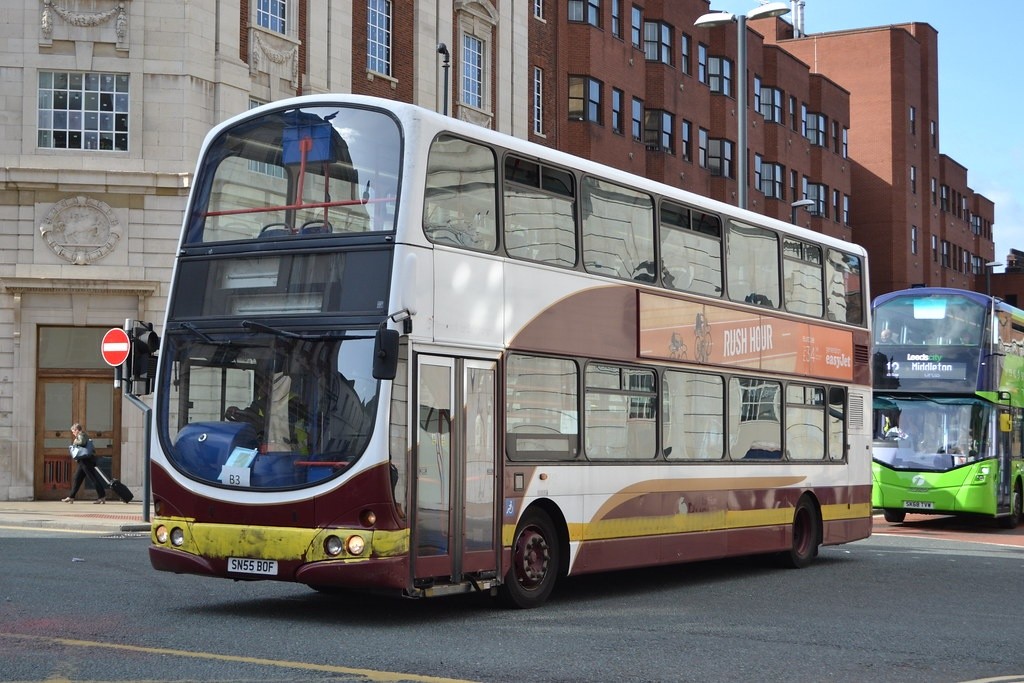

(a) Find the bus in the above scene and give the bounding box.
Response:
[871,287,1024,529]
[145,94,875,609]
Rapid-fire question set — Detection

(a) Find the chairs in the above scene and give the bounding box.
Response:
[257,223,293,237]
[300,219,332,234]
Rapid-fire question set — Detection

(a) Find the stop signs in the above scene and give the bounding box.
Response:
[101,328,131,367]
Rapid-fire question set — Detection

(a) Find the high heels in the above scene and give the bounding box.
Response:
[61,497,75,504]
[93,497,105,504]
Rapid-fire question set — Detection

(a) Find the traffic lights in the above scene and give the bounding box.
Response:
[135,327,162,355]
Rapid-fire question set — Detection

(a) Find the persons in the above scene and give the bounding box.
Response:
[62,422,106,504]
[877,328,892,344]
[884,413,917,444]
[225,371,309,455]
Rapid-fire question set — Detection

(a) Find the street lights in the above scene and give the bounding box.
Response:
[791,199,815,225]
[984,261,1003,296]
[693,2,792,209]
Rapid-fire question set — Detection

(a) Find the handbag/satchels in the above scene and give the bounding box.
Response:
[69,433,93,459]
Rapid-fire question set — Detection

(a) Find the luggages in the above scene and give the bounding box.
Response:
[93,463,134,503]
